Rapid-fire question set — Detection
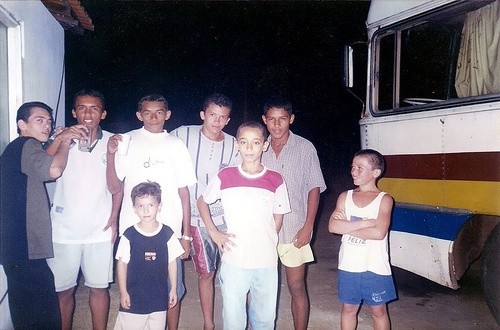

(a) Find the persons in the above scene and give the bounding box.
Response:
[106,95,193,330]
[44,90,124,330]
[0,101,71,330]
[170,94,242,330]
[247,100,326,330]
[114,182,184,330]
[197,121,292,330]
[328,149,397,330]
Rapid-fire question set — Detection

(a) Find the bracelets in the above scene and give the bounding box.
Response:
[180,236,194,241]
[202,132,221,140]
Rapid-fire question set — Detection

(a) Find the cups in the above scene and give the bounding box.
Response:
[78,126,91,151]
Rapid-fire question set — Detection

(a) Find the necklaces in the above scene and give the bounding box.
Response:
[271,138,287,153]
[138,130,167,167]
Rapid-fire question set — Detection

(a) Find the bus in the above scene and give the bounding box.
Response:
[341,0,500,326]
